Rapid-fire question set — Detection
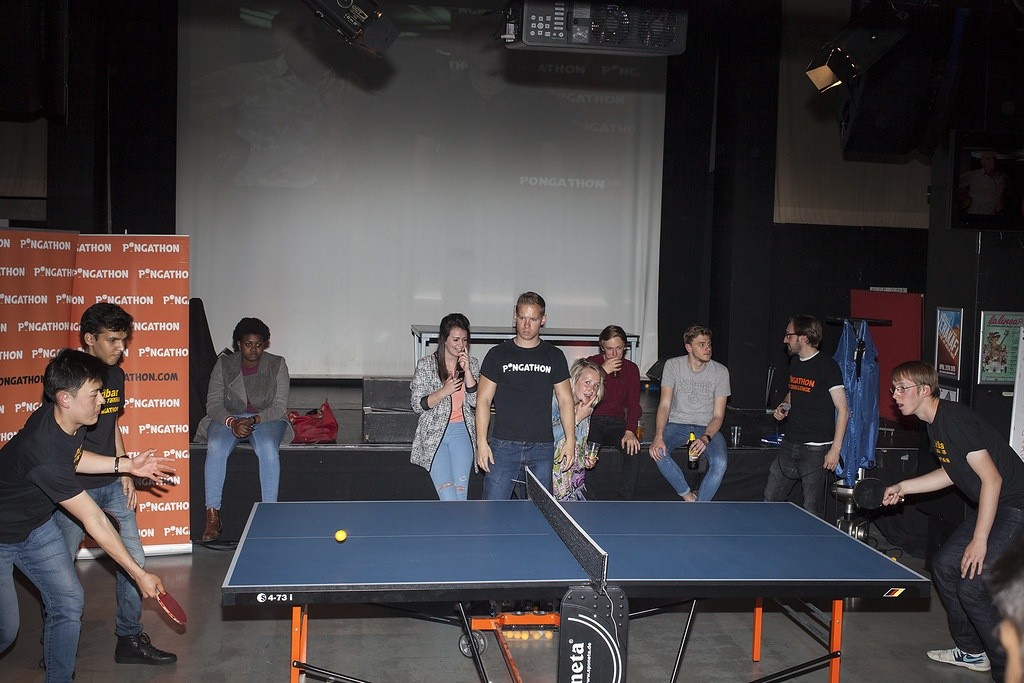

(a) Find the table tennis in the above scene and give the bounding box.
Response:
[514,632,521,639]
[891,557,896,561]
[522,633,529,640]
[545,632,553,639]
[335,530,347,541]
[533,632,540,640]
[506,631,513,639]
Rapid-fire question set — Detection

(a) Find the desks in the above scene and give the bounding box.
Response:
[409,324,639,383]
[222,467,929,683]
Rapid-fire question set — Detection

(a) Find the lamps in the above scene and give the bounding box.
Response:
[802,1,912,94]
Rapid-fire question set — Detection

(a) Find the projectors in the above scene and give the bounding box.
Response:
[502,0,688,56]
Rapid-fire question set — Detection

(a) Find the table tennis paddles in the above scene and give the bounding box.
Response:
[156,587,187,625]
[853,478,905,509]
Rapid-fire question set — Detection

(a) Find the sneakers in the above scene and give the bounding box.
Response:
[115,632,177,664]
[927,647,991,671]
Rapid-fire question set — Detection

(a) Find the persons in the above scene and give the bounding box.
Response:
[193,317,294,542]
[53,303,178,665]
[476,292,575,500]
[882,360,1024,683]
[649,328,731,501]
[410,314,479,501]
[987,541,1024,683]
[0,349,177,683]
[552,359,602,502]
[765,315,850,520]
[585,325,643,501]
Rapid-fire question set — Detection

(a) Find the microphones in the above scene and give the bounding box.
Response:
[781,402,792,414]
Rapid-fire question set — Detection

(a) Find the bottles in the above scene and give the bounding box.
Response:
[687,431,699,470]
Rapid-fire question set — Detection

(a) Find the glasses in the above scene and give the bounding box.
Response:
[784,332,808,339]
[890,382,932,393]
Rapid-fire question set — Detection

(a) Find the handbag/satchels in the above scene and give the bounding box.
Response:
[288,402,338,445]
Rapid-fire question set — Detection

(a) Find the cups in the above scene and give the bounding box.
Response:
[636,421,646,443]
[587,441,601,459]
[731,425,742,445]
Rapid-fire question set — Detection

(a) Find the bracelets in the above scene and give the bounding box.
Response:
[115,457,119,474]
[252,416,256,424]
[121,455,129,458]
[703,434,711,443]
[225,416,235,428]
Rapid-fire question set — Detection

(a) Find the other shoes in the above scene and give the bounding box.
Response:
[453,601,474,612]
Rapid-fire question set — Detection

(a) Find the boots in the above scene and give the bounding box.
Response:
[202,507,223,541]
